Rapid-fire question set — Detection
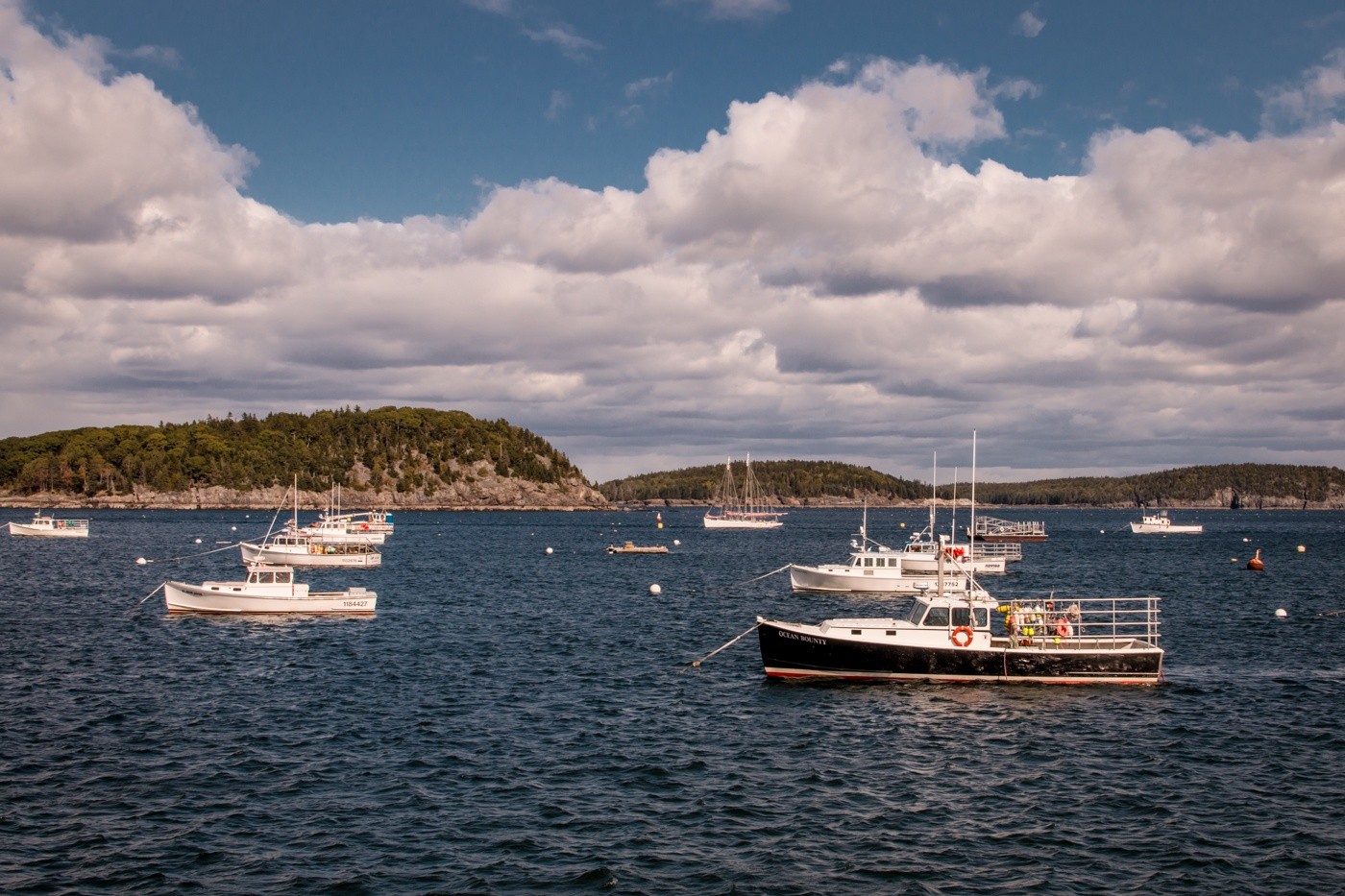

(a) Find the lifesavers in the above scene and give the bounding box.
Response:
[953,626,974,647]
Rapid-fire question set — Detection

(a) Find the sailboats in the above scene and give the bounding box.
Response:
[722,452,789,516]
[702,453,784,528]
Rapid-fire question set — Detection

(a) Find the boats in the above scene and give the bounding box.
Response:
[966,512,1051,542]
[237,468,398,575]
[161,557,380,618]
[784,493,968,595]
[887,448,1007,572]
[1130,500,1205,535]
[755,426,1166,686]
[8,507,91,538]
